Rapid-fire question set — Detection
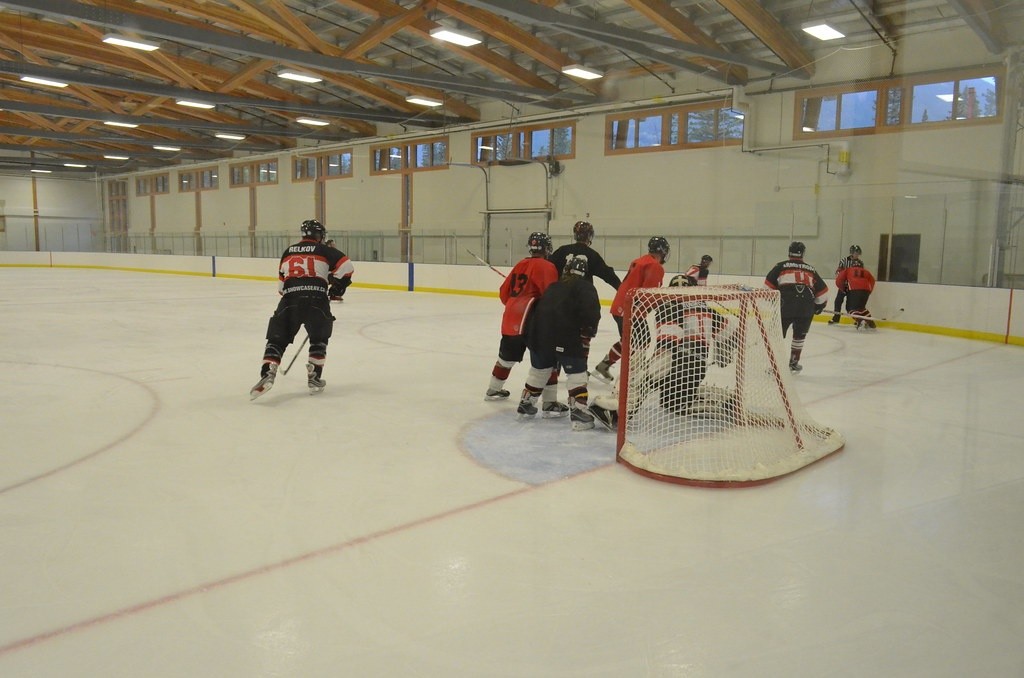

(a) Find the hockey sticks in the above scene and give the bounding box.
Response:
[822,308,906,322]
[611,361,716,395]
[713,301,750,318]
[451,230,508,279]
[278,298,333,376]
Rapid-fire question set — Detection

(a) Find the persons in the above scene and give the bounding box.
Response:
[836,262,877,331]
[484,231,569,419]
[687,255,712,286]
[589,274,738,432]
[250,219,355,401]
[516,256,596,430]
[592,236,670,384]
[548,220,622,375]
[765,241,828,374]
[828,245,864,325]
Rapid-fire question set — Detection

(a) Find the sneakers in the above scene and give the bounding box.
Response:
[788,360,803,375]
[865,322,877,330]
[586,396,620,433]
[856,319,866,332]
[249,372,277,400]
[541,401,571,421]
[828,319,840,325]
[516,398,538,421]
[570,407,596,431]
[485,387,510,401]
[591,362,615,385]
[308,373,326,395]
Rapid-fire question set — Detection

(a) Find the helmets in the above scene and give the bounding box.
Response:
[702,255,713,263]
[647,235,671,257]
[301,219,327,242]
[528,231,554,256]
[849,245,864,256]
[669,275,690,287]
[573,221,595,244]
[788,240,805,256]
